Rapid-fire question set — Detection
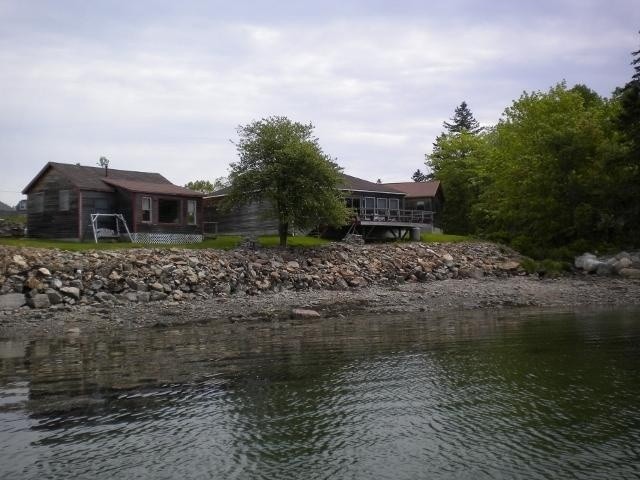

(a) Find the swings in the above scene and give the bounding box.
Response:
[94,215,120,239]
